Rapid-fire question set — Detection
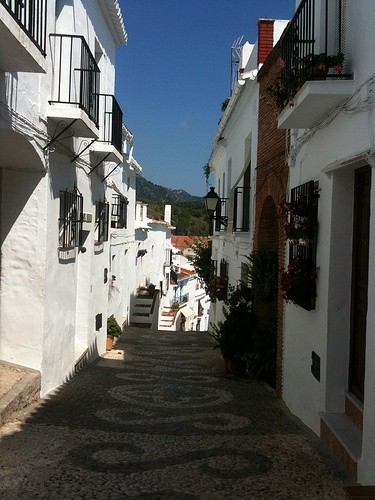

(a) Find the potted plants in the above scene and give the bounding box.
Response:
[106,314,122,352]
[209,303,269,382]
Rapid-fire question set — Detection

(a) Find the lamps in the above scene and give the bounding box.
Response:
[203,187,228,227]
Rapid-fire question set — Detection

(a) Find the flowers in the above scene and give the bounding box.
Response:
[278,256,317,312]
[262,51,345,112]
[277,192,314,246]
[206,276,228,302]
